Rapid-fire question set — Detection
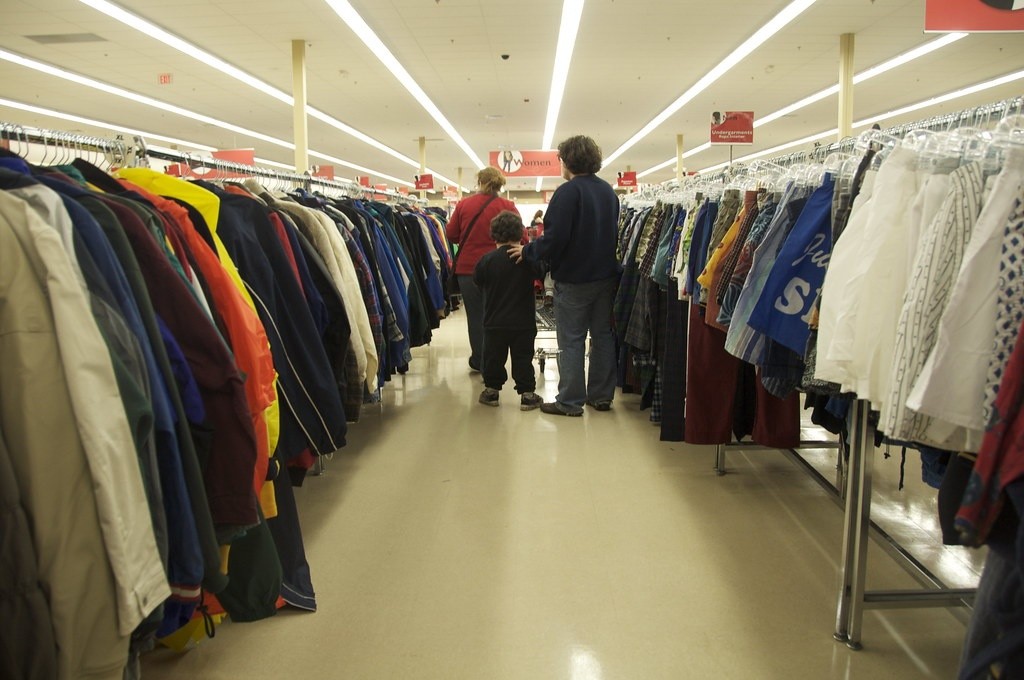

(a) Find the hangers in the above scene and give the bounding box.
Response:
[0,96,1024,214]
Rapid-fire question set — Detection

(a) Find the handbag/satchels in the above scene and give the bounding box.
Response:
[445,267,461,295]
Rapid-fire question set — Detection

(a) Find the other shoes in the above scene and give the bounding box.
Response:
[520,392,544,410]
[469,356,481,373]
[587,401,611,411]
[478,389,499,406]
[540,402,583,416]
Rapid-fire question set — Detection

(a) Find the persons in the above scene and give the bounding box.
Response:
[531,210,543,226]
[472,210,544,411]
[445,166,530,371]
[507,135,625,416]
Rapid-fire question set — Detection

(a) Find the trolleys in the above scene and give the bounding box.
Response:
[532,289,590,372]
